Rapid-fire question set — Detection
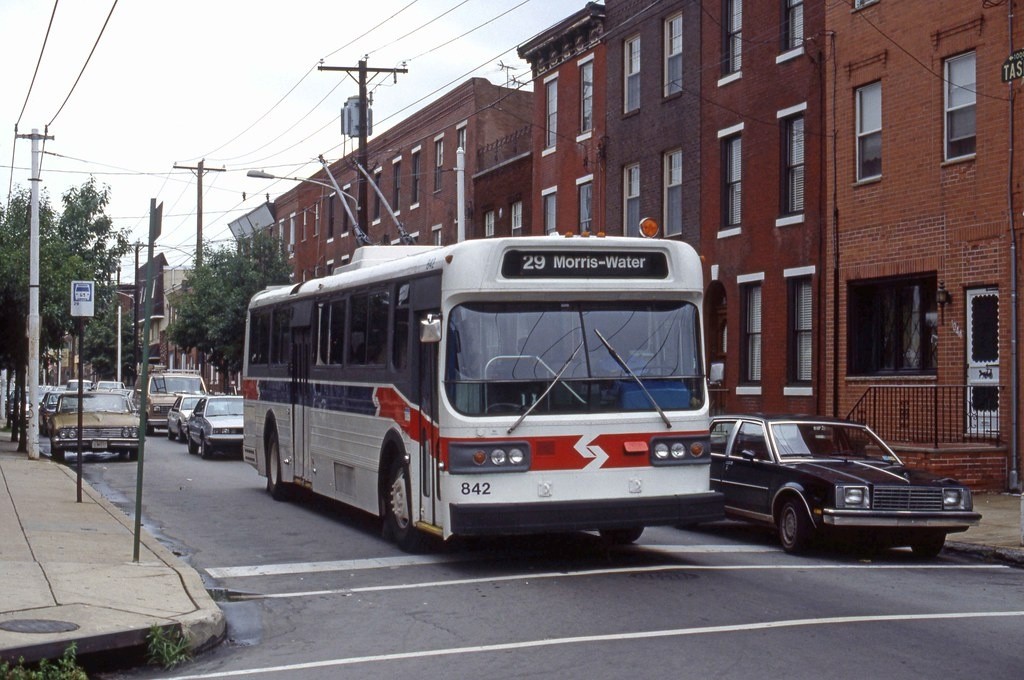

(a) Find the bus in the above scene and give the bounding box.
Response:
[241,214,713,552]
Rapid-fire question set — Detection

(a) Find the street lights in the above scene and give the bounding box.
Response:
[242,169,372,249]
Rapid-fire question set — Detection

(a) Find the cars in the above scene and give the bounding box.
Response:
[1,370,208,435]
[184,396,245,459]
[167,394,205,444]
[50,392,141,462]
[672,414,983,560]
